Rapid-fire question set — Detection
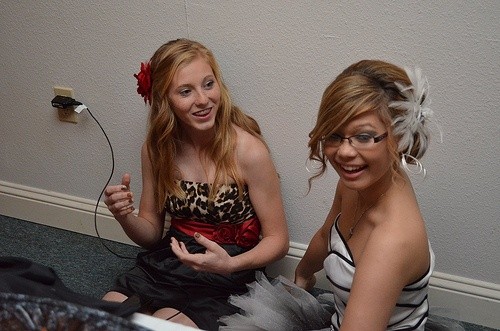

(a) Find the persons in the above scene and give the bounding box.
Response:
[103,38,290,331]
[290,58,436,331]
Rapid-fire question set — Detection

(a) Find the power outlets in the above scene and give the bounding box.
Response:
[54,87,77,123]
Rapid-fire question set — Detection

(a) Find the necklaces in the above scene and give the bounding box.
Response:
[345,185,387,242]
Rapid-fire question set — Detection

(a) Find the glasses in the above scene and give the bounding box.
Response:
[321,131,388,146]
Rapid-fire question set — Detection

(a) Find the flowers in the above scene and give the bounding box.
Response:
[133,61,153,106]
